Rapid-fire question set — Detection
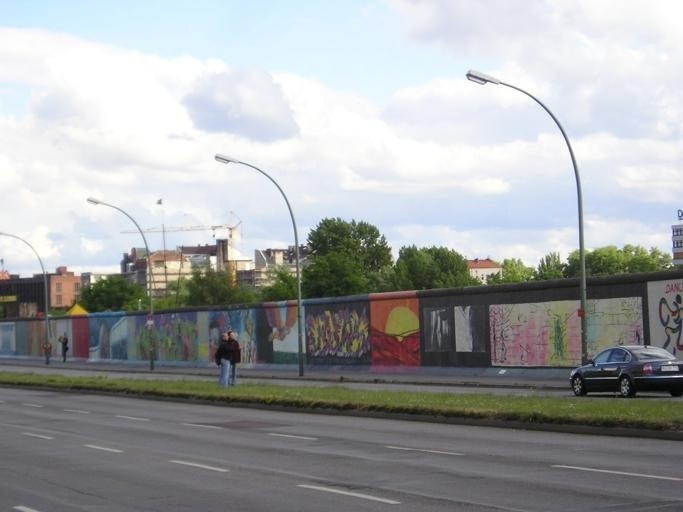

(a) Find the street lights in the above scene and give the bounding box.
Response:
[463,68,589,367]
[212,152,304,379]
[85,197,155,370]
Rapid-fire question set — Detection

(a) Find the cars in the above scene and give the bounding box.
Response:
[570,344,682,398]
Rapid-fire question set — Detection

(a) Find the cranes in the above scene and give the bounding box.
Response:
[117,222,244,252]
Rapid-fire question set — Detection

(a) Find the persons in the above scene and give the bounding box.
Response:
[57,332,68,363]
[225,331,240,385]
[216,332,233,388]
